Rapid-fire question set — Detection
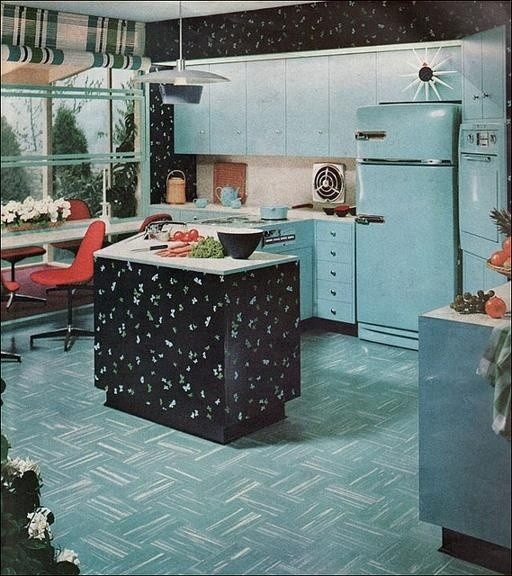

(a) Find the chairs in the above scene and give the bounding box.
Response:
[59,197,112,256]
[1,245,47,310]
[137,212,171,235]
[28,217,110,351]
[0,266,23,366]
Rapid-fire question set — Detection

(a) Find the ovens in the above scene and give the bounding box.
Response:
[458,120,508,263]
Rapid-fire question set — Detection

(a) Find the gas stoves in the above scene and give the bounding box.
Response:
[186,213,306,229]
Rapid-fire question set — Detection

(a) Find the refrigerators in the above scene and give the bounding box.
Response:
[354,101,463,350]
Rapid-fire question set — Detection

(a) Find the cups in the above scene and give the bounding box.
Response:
[231,197,242,209]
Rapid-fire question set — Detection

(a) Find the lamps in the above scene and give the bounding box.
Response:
[132,1,230,89]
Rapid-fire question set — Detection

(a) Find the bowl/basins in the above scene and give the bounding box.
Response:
[322,205,356,216]
[217,229,263,259]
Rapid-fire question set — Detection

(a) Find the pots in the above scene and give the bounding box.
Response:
[260,202,313,220]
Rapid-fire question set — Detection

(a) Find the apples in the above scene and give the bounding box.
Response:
[490,237,512,270]
[485,297,506,318]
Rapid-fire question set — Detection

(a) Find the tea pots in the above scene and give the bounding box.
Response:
[166,169,187,204]
[193,198,209,208]
[215,186,241,208]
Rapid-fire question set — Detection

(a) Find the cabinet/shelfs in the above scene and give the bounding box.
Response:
[175,46,463,161]
[460,26,507,120]
[459,251,510,299]
[312,216,357,331]
[149,205,237,221]
[417,280,512,574]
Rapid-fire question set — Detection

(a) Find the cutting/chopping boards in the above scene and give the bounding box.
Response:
[213,161,247,206]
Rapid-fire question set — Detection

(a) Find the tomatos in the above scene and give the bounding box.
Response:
[174,229,204,242]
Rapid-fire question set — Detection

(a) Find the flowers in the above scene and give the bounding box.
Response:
[1,430,85,576]
[0,196,74,231]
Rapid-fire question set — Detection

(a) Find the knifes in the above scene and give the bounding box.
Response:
[129,244,168,252]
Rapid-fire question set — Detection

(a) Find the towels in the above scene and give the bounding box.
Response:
[475,318,512,438]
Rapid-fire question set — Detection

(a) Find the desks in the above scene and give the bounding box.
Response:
[1,217,147,251]
